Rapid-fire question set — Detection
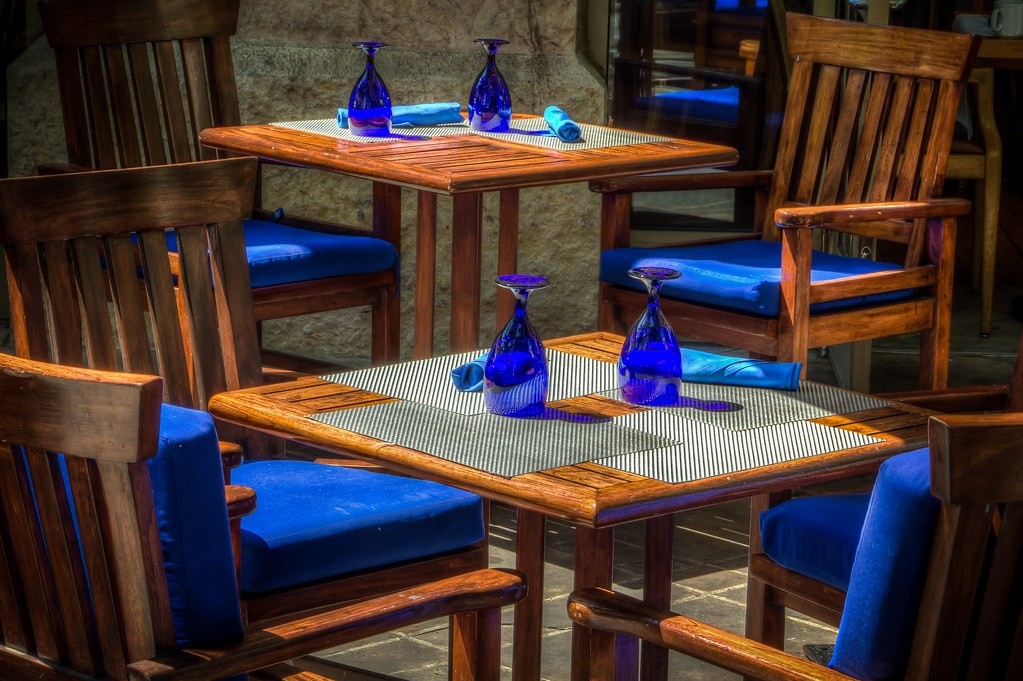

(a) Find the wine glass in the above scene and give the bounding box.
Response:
[616,266,682,406]
[467,39,512,132]
[482,275,551,418]
[349,41,393,136]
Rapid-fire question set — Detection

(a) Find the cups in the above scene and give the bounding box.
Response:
[990,0,1023,40]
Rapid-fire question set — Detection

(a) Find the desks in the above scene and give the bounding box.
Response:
[209,331,947,680]
[198,111,739,362]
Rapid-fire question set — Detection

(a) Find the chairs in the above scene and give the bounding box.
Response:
[0,0,1023,681]
